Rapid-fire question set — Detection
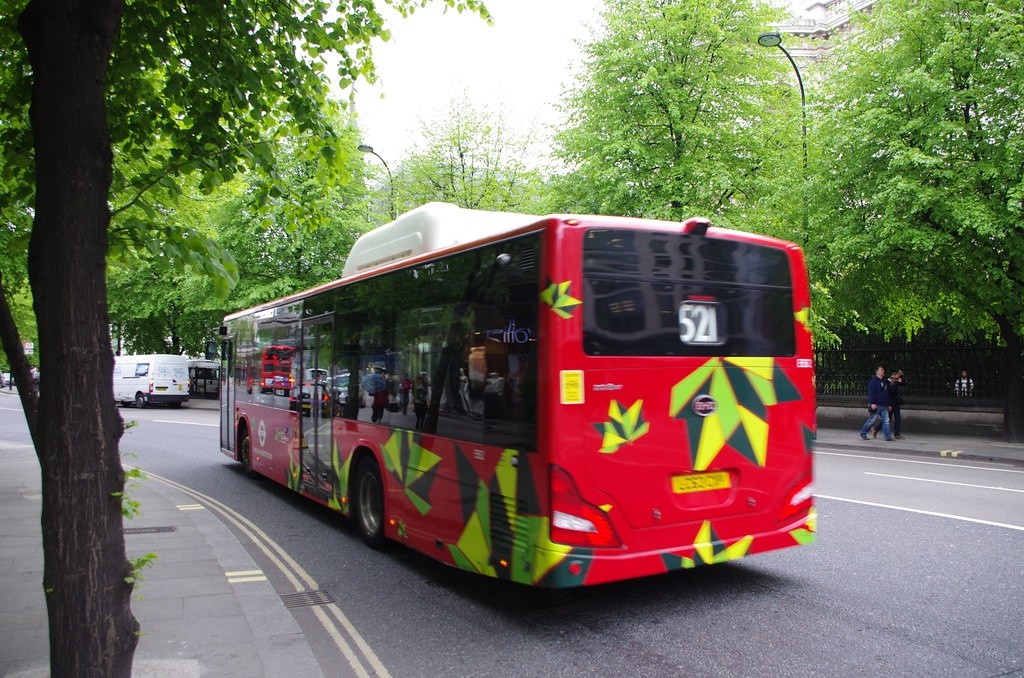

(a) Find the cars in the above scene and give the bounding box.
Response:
[289,367,366,420]
[0,371,16,389]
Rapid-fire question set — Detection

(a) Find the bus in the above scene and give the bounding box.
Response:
[258,344,296,397]
[204,200,820,590]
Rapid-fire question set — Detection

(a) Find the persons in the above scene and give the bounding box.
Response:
[955,369,974,399]
[869,371,906,440]
[887,367,904,386]
[858,365,896,443]
[246,359,505,432]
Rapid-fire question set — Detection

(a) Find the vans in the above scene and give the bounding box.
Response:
[111,353,191,409]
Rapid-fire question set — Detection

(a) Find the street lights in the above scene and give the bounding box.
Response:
[757,32,812,254]
[356,145,397,220]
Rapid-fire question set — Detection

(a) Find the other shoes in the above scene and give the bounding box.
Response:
[894,435,905,440]
[860,435,870,440]
[871,427,877,438]
[887,438,896,441]
